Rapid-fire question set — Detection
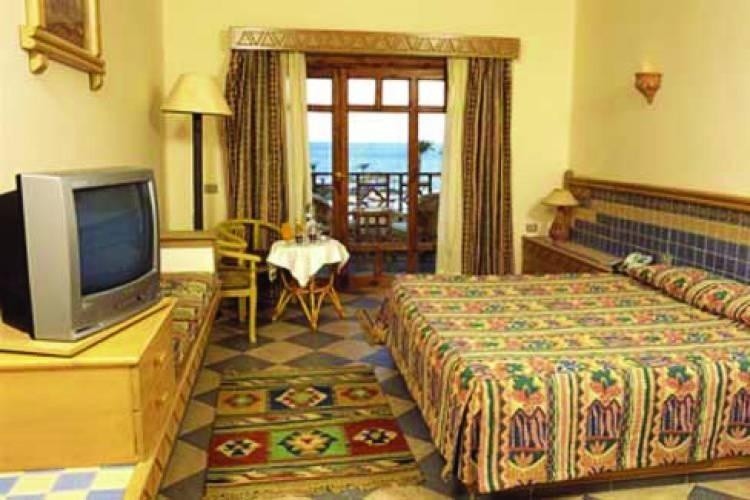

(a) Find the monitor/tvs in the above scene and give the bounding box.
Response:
[0,165,160,341]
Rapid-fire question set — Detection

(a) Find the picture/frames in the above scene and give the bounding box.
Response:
[19,0,106,90]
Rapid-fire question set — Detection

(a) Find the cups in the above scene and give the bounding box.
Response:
[319,223,330,239]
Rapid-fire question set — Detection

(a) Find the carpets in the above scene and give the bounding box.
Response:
[206,363,424,500]
[354,307,386,345]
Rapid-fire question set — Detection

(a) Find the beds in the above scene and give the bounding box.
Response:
[377,265,750,500]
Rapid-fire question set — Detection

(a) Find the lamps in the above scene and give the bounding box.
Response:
[159,73,232,231]
[540,189,579,243]
[633,64,662,104]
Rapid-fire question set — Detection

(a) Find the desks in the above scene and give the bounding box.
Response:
[266,237,350,332]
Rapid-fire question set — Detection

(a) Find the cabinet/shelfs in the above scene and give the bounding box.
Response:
[0,298,178,472]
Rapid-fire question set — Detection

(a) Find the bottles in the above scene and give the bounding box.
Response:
[295,218,304,246]
[307,214,316,243]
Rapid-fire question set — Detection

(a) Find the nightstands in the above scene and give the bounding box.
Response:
[523,236,621,275]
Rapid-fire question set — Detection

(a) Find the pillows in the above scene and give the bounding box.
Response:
[685,279,750,325]
[627,263,719,300]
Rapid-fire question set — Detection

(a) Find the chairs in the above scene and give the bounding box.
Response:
[215,219,282,322]
[215,240,262,344]
